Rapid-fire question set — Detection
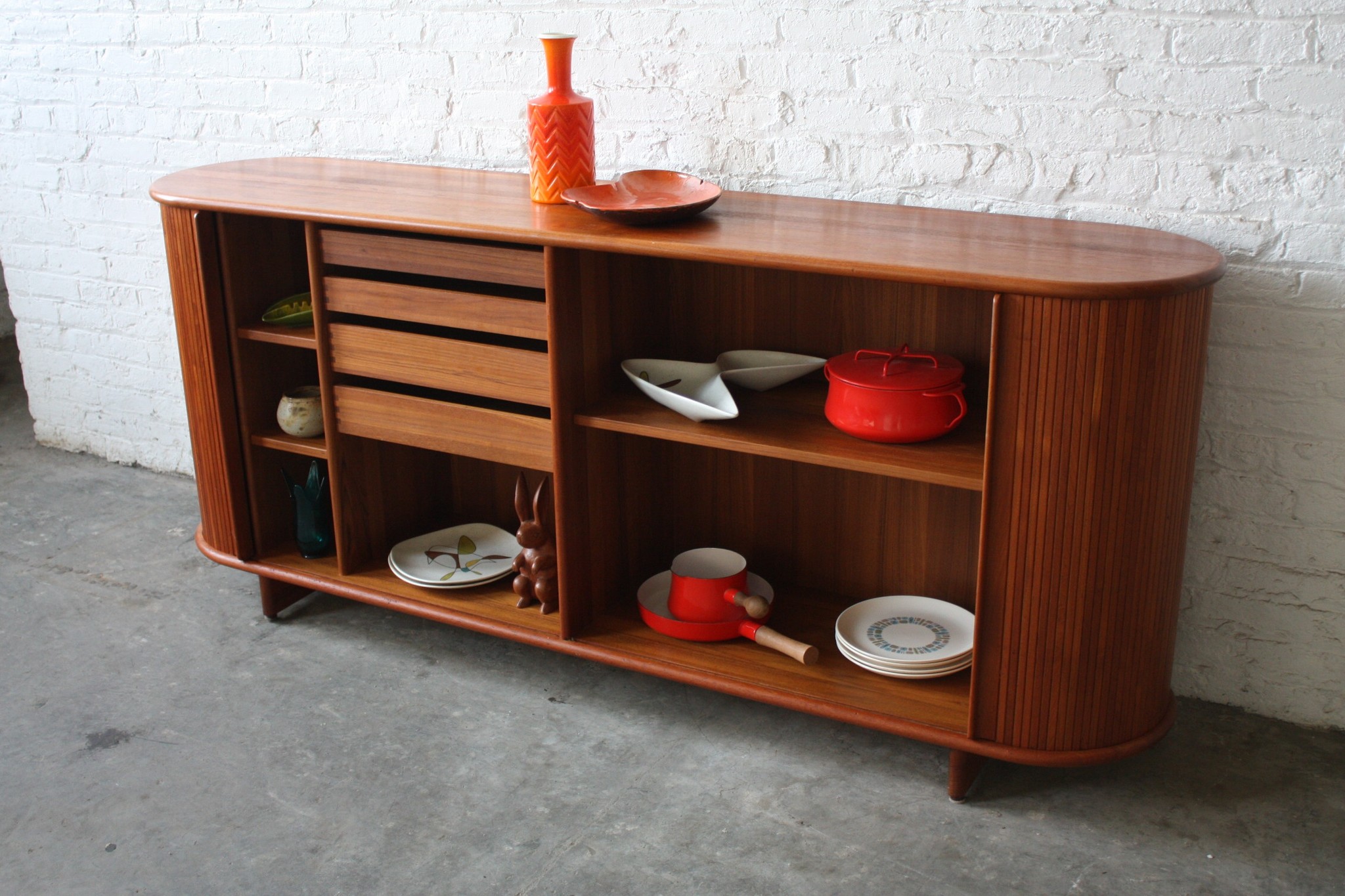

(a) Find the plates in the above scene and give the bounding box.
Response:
[834,594,974,678]
[387,521,523,589]
[561,169,722,222]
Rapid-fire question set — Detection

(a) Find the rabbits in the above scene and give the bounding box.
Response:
[512,471,558,614]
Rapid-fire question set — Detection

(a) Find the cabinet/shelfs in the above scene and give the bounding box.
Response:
[152,155,1228,805]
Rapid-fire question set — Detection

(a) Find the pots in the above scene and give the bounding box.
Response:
[636,568,821,666]
[824,344,968,444]
[666,545,770,623]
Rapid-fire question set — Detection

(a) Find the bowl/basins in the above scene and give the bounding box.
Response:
[262,292,313,324]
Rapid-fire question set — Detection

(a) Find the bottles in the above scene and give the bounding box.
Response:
[528,31,596,205]
[280,460,330,558]
[276,385,324,438]
